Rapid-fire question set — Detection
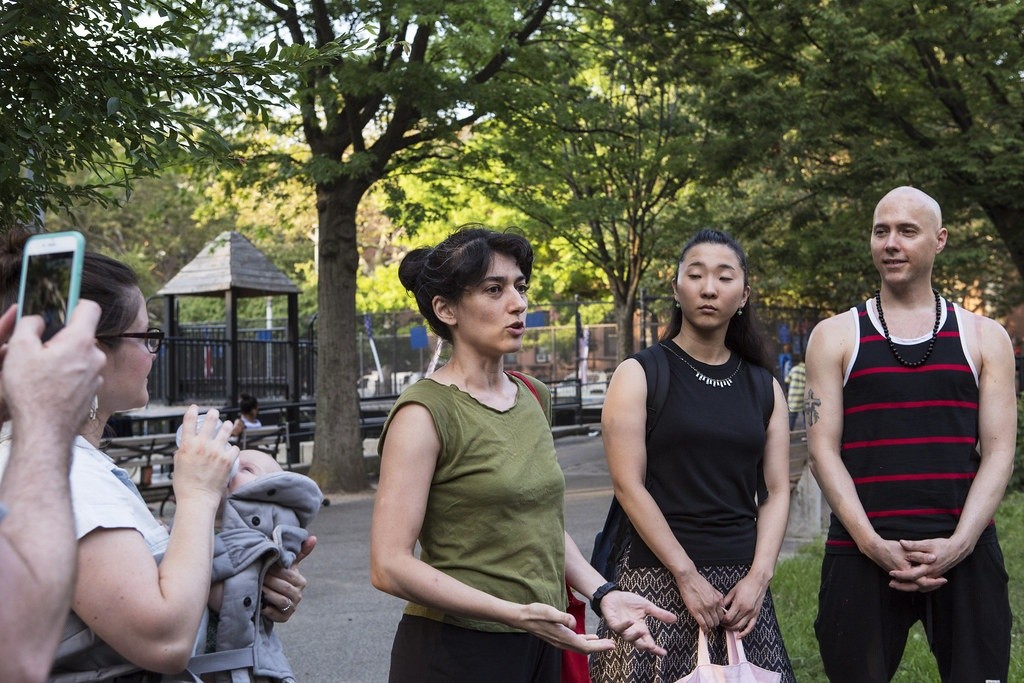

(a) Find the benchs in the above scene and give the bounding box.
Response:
[100,423,292,518]
[788,428,809,485]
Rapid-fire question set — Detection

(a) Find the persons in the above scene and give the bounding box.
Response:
[781,185,1024,683]
[0,224,319,683]
[370,224,678,683]
[588,228,798,683]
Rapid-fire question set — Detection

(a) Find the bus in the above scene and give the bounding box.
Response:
[551,371,612,398]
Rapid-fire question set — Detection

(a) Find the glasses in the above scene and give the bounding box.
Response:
[94,327,166,353]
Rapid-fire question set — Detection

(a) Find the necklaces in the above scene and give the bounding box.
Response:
[660,341,744,388]
[874,287,942,366]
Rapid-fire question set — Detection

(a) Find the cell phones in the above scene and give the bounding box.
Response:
[16,231,86,342]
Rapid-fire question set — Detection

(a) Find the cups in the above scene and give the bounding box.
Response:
[141,465,153,485]
[161,463,173,473]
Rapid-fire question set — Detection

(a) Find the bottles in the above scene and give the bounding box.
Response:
[175,414,240,483]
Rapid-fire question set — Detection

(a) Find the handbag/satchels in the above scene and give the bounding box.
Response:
[672,607,782,683]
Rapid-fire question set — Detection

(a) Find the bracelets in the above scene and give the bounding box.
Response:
[591,583,623,620]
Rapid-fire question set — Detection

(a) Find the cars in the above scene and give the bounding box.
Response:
[357,371,421,399]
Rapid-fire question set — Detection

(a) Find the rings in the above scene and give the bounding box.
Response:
[280,599,292,613]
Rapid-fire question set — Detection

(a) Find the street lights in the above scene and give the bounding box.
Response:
[567,287,596,426]
